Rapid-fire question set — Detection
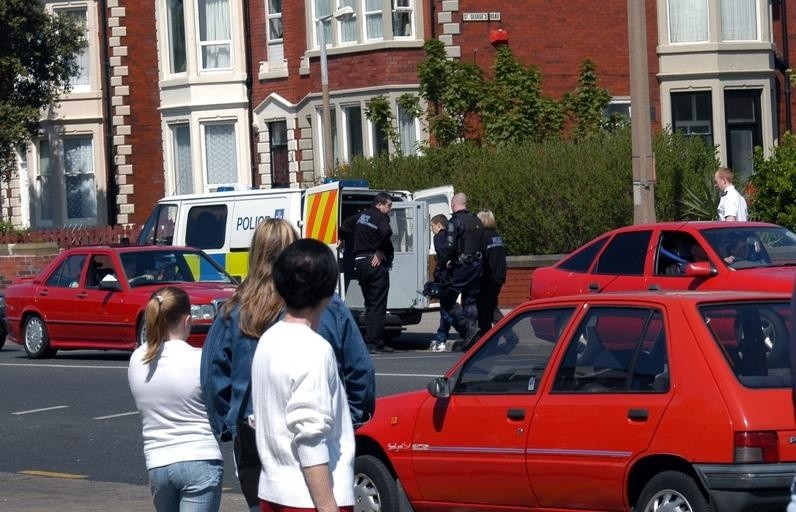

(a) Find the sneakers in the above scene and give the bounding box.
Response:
[428,340,446,352]
[462,328,519,350]
[367,344,393,354]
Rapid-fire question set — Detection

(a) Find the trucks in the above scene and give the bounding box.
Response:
[121,176,454,342]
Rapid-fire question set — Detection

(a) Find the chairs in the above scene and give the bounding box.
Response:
[665,243,693,276]
[584,351,665,389]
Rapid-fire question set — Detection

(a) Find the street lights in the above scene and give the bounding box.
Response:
[312,5,354,179]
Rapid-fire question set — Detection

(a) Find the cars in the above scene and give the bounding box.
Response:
[351,296,796,512]
[528,215,795,369]
[2,244,242,357]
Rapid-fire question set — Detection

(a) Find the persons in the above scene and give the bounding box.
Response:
[665,232,738,278]
[427,214,459,352]
[198,217,378,511]
[336,191,395,353]
[125,285,227,510]
[438,191,485,354]
[249,237,359,510]
[68,254,180,289]
[475,207,519,355]
[714,166,750,221]
